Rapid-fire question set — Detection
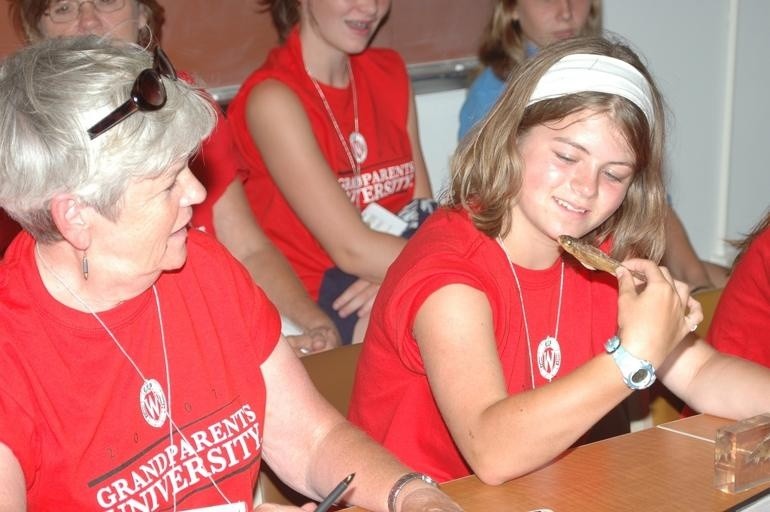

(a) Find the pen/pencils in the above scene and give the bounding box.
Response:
[314,473,355,512]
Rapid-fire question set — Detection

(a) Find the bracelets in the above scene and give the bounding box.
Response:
[386,468,440,510]
[689,282,715,294]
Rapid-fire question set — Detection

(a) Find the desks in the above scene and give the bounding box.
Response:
[328,410,770,511]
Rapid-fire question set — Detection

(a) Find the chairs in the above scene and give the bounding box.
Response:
[652,286,732,426]
[260,338,361,504]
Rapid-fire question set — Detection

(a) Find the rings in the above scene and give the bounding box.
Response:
[684,317,699,334]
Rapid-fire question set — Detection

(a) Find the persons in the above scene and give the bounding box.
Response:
[346,32,770,490]
[6,0,343,362]
[228,1,450,347]
[1,35,475,512]
[458,1,720,296]
[678,212,769,424]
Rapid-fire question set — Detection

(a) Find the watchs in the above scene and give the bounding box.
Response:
[603,334,657,400]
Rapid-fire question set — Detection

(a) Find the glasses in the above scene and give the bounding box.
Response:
[40,0,125,22]
[90,45,177,141]
